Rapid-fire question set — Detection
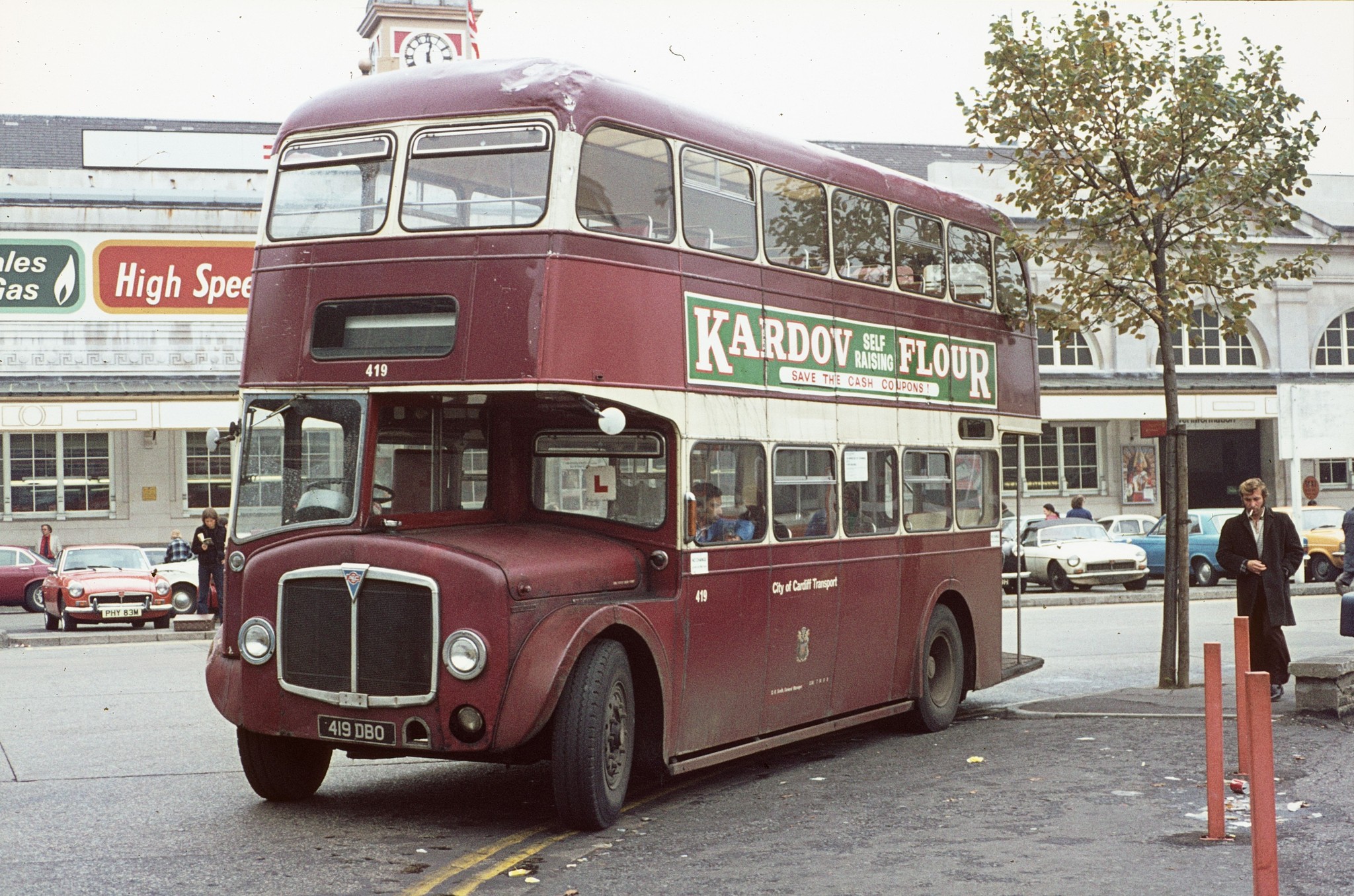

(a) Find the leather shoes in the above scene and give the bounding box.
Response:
[1270,684,1284,700]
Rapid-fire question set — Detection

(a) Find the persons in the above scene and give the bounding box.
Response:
[1043,503,1060,520]
[1215,478,1304,701]
[1066,495,1093,521]
[35,524,63,562]
[843,482,876,534]
[692,483,755,543]
[994,501,1015,519]
[164,529,193,563]
[191,508,228,626]
[1334,505,1354,596]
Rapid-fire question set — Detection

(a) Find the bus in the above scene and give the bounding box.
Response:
[203,57,1046,833]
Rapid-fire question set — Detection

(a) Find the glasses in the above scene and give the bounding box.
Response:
[41,529,49,531]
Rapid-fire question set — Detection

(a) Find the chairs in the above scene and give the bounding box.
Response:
[844,517,875,534]
[108,560,136,568]
[752,518,791,539]
[66,562,86,570]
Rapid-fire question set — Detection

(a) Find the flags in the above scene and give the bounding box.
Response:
[468,0,480,59]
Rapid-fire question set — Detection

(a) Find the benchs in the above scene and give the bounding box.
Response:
[714,235,756,257]
[578,213,653,239]
[924,280,957,300]
[948,284,987,305]
[766,246,809,270]
[652,225,714,251]
[850,264,886,285]
[812,256,849,277]
[904,507,981,531]
[897,273,925,294]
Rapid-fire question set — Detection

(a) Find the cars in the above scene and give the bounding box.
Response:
[1112,508,1245,586]
[41,544,173,631]
[1268,506,1347,581]
[1002,517,1150,593]
[1000,515,1067,543]
[1098,514,1160,539]
[0,545,54,612]
[150,555,199,614]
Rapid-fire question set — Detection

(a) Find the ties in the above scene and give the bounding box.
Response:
[44,537,48,556]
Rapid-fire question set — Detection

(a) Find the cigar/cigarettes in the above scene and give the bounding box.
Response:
[1249,509,1253,515]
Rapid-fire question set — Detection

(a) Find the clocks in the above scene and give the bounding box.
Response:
[404,33,452,67]
